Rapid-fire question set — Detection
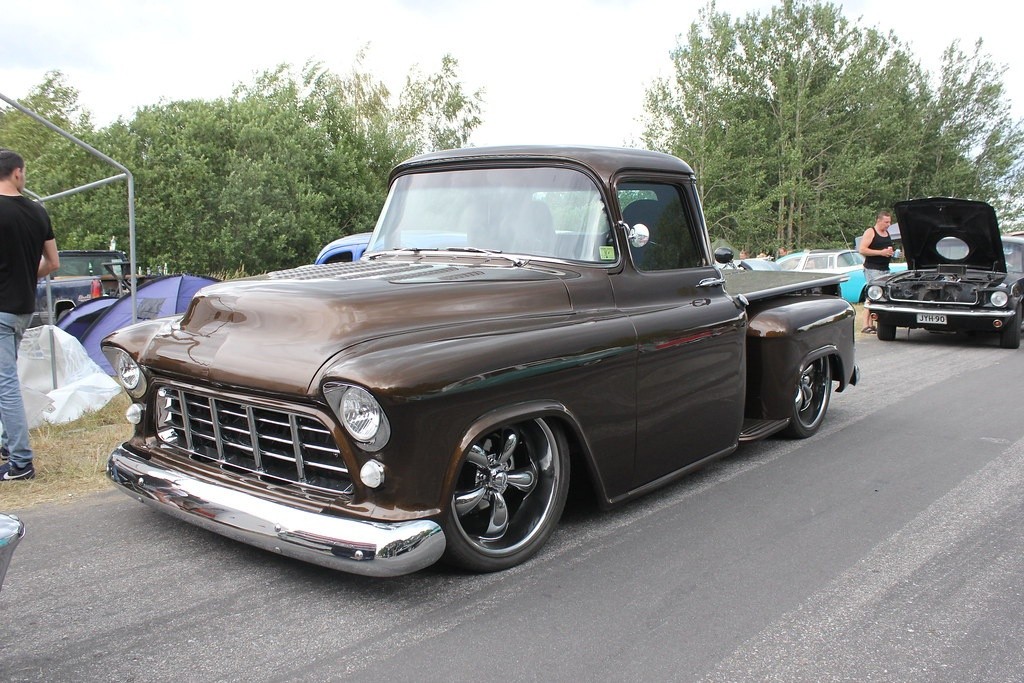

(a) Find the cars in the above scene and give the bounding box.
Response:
[861,195,1024,347]
[314,229,783,274]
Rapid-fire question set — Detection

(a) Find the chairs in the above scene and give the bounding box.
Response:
[619,200,687,272]
[499,200,556,254]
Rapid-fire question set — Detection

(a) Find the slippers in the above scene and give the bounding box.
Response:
[860,326,878,334]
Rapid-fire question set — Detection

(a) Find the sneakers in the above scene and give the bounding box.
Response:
[0,447,10,460]
[0,461,36,481]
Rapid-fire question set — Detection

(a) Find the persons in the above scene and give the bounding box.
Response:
[859,210,903,334]
[739,248,788,270]
[0,151,59,481]
[893,246,905,263]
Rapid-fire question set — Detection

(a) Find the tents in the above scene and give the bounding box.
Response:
[855,222,903,252]
[56,272,223,377]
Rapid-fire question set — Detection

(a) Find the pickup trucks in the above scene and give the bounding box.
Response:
[105,145,856,581]
[776,248,907,305]
[34,251,134,325]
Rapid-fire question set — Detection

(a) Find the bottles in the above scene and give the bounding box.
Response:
[89,262,94,276]
[164,263,168,275]
[138,266,142,275]
[109,235,116,252]
